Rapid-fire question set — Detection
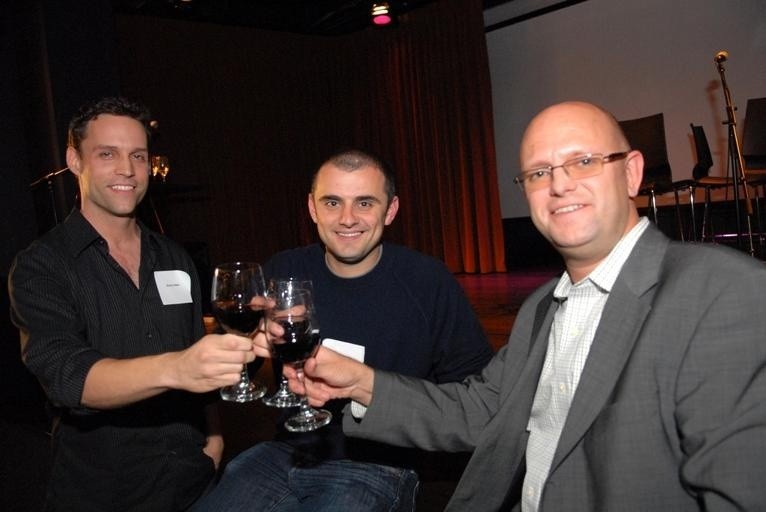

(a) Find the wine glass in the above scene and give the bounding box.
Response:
[210,260,271,403]
[262,277,333,435]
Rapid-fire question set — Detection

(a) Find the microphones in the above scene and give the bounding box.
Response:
[149,120,160,129]
[714,50,729,63]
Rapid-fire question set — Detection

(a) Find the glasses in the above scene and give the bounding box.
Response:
[512,150,628,192]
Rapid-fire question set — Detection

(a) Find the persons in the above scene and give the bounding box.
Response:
[178,144,496,511]
[280,95,764,510]
[5,94,257,511]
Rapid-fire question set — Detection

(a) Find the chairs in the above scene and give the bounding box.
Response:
[614,97,766,246]
